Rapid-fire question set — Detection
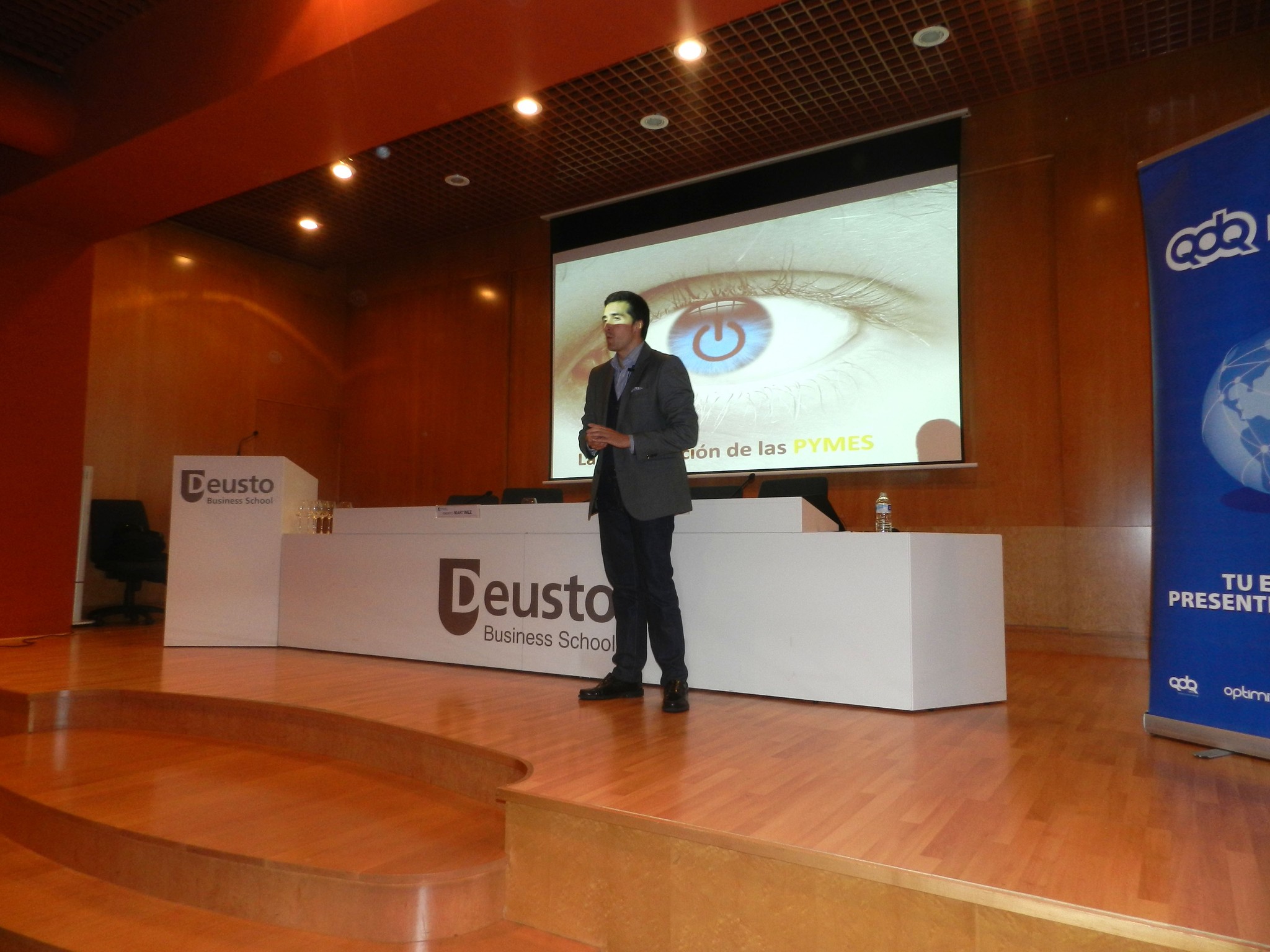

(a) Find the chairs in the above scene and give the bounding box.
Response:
[86,499,169,626]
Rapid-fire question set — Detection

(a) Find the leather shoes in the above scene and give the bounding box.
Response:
[579,671,644,699]
[662,680,690,713]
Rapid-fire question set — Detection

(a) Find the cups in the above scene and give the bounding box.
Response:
[522,498,538,504]
[338,501,353,508]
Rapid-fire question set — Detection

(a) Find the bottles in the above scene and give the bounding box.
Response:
[875,492,892,533]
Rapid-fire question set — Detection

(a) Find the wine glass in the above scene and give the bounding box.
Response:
[294,499,337,534]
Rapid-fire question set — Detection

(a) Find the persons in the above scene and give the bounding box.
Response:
[579,291,699,714]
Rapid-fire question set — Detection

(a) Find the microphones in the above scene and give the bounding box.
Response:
[237,430,259,455]
[627,368,635,372]
